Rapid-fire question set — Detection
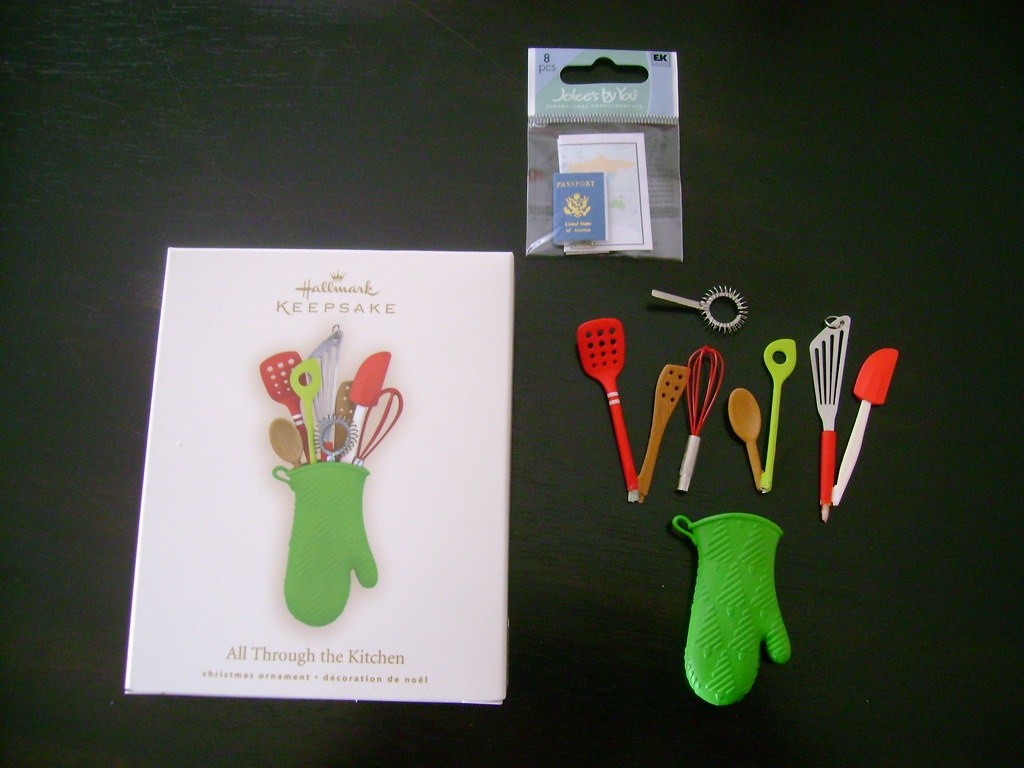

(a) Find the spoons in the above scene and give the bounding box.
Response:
[727,388,763,490]
[270,417,303,468]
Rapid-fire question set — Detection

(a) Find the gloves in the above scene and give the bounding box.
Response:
[272,463,378,627]
[671,513,791,705]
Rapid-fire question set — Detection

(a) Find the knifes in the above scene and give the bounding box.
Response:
[832,348,899,506]
[341,351,392,466]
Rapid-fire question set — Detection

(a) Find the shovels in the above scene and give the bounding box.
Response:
[576,316,694,504]
[810,314,852,525]
